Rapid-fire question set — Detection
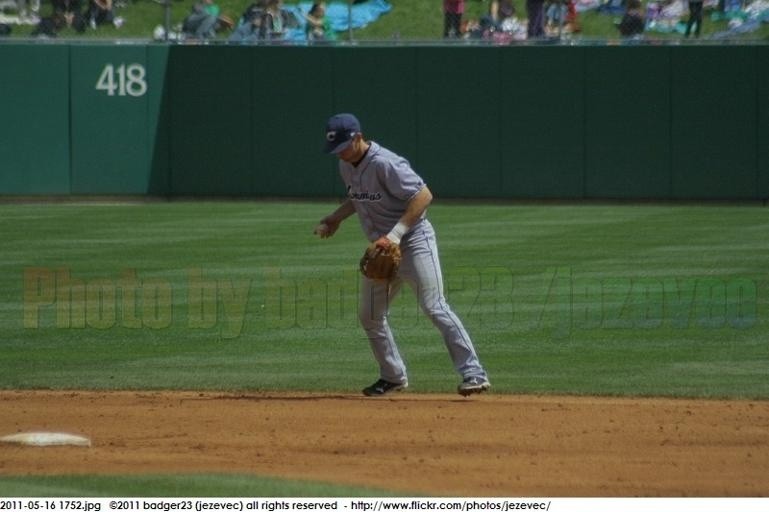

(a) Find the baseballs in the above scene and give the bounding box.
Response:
[316,224,329,232]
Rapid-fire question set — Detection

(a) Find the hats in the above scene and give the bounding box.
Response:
[320,112,362,157]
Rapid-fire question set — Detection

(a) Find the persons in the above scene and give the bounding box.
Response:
[310,111,492,396]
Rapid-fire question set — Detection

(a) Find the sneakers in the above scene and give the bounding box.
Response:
[362,375,410,398]
[456,370,490,397]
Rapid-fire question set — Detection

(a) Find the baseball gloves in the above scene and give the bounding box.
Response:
[360,235,402,281]
